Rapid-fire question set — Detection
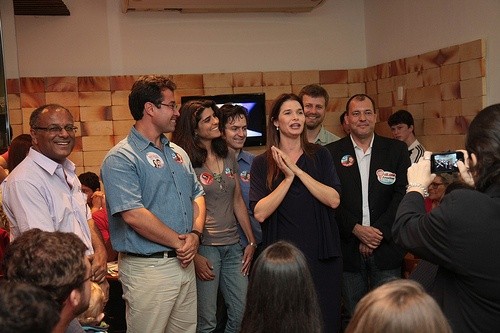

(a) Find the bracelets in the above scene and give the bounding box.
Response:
[248,242,257,248]
[405,183,429,199]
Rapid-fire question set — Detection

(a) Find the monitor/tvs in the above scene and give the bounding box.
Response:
[179,92,267,148]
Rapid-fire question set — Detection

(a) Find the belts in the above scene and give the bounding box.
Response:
[122,251,177,259]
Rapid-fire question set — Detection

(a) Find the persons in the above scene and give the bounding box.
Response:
[166,100,257,333]
[0,103,126,333]
[99,74,208,333]
[237,238,325,333]
[0,229,91,333]
[433,156,458,171]
[392,102,500,333]
[246,83,429,333]
[341,280,456,332]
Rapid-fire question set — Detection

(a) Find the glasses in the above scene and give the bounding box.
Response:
[33,125,78,134]
[157,102,178,112]
[81,270,96,285]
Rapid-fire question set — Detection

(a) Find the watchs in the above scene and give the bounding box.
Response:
[190,229,205,245]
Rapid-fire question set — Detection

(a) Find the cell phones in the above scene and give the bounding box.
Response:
[431,151,464,173]
